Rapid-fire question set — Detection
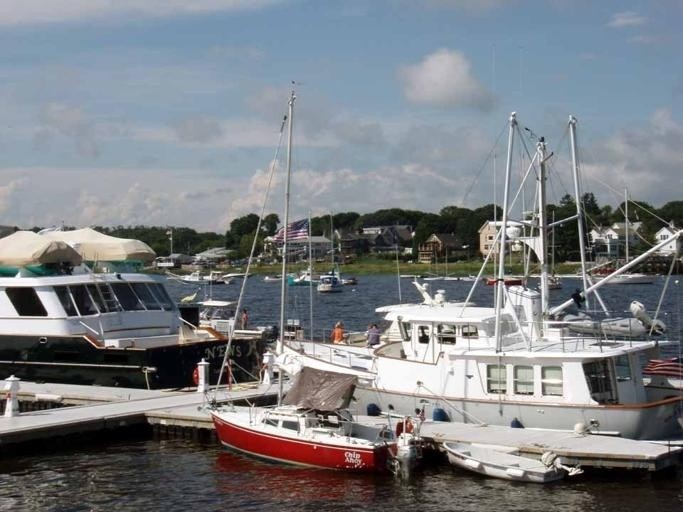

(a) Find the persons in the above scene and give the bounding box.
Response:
[406,416,414,435]
[241,308,250,330]
[415,403,426,423]
[332,320,348,346]
[366,324,382,347]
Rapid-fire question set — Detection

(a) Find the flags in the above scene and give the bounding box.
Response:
[263,218,308,245]
[642,356,683,378]
